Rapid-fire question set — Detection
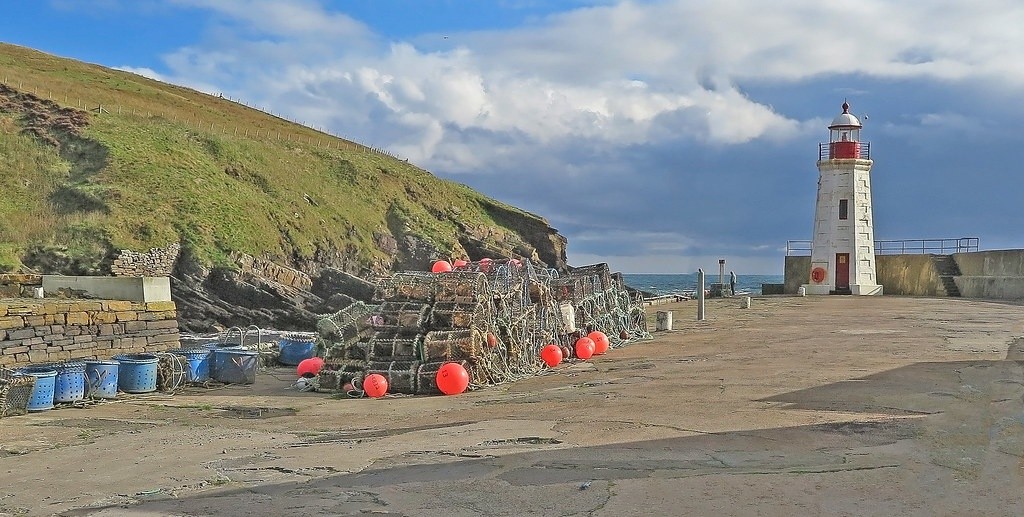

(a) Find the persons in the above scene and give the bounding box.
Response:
[842,133,847,142]
[730,271,736,295]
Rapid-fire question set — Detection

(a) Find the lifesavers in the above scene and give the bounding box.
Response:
[812,268,823,282]
